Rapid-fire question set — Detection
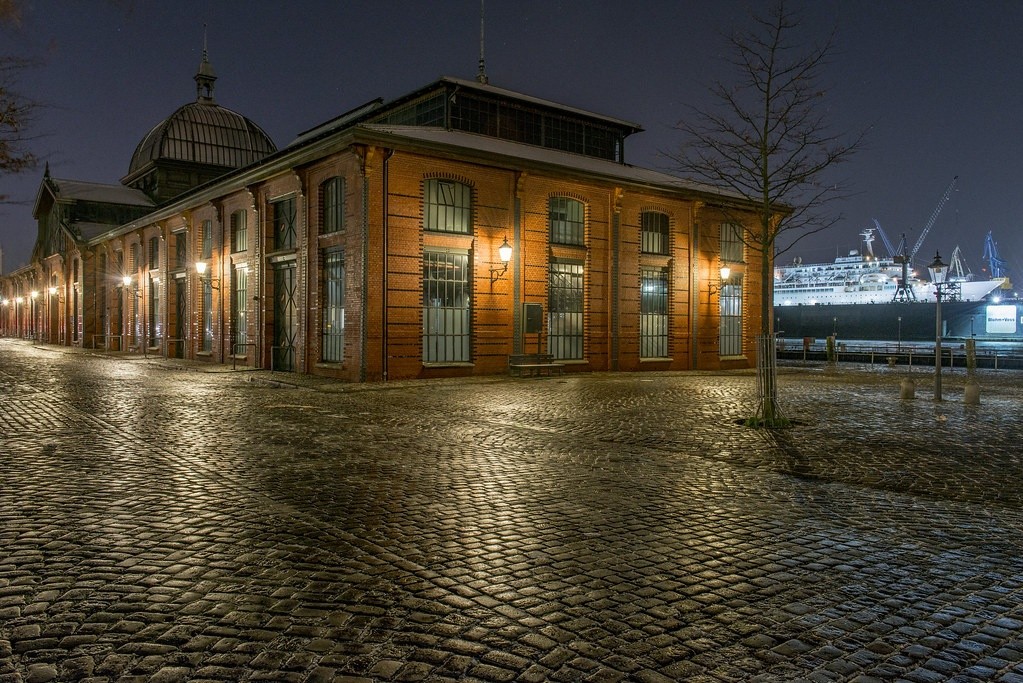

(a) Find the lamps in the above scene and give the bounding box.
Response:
[708,262,731,296]
[489,235,512,287]
[123,272,143,299]
[196,254,220,292]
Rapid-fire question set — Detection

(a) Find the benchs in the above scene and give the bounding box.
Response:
[506,353,565,378]
